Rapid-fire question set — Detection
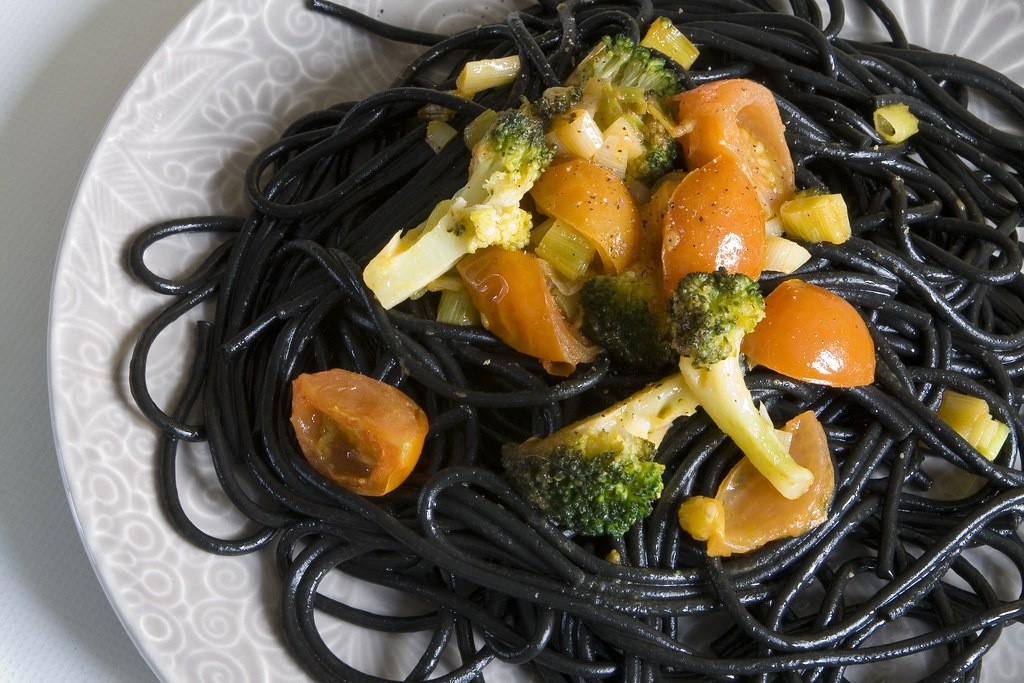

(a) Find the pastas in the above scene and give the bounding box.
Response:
[126,0,1024,682]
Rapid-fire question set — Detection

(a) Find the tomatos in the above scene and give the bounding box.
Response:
[291,368,429,495]
[741,280,877,386]
[675,77,795,221]
[527,157,645,278]
[455,246,611,376]
[661,156,766,307]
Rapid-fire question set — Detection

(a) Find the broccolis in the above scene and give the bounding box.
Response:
[669,268,811,502]
[580,268,674,374]
[572,35,684,183]
[499,381,700,536]
[364,114,553,310]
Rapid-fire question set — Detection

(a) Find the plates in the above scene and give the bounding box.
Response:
[48,2,1024,683]
